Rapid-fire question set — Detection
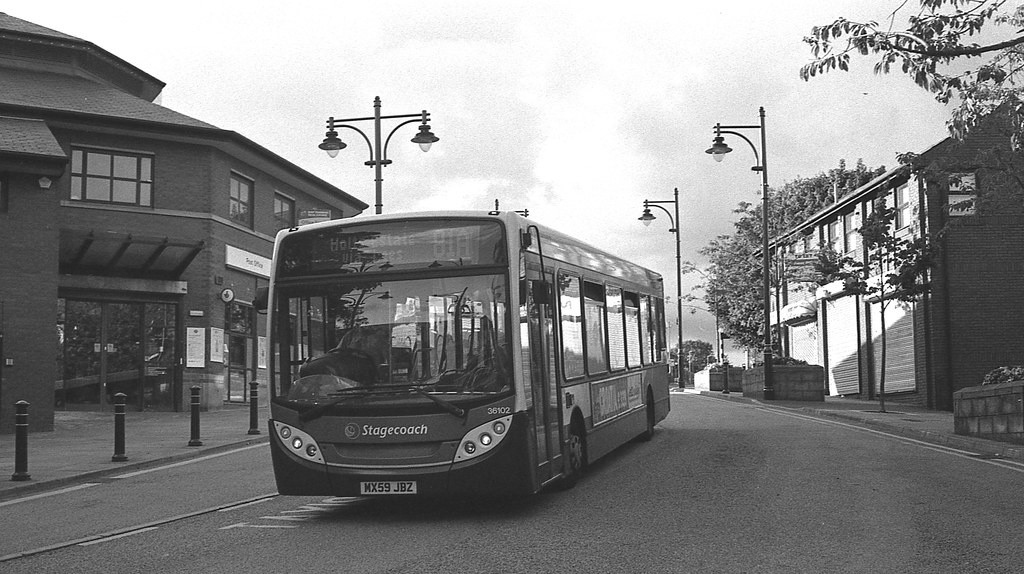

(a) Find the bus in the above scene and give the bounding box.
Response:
[250,211,672,500]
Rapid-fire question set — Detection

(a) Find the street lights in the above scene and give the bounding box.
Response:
[703,123,774,402]
[318,110,440,214]
[637,199,686,388]
